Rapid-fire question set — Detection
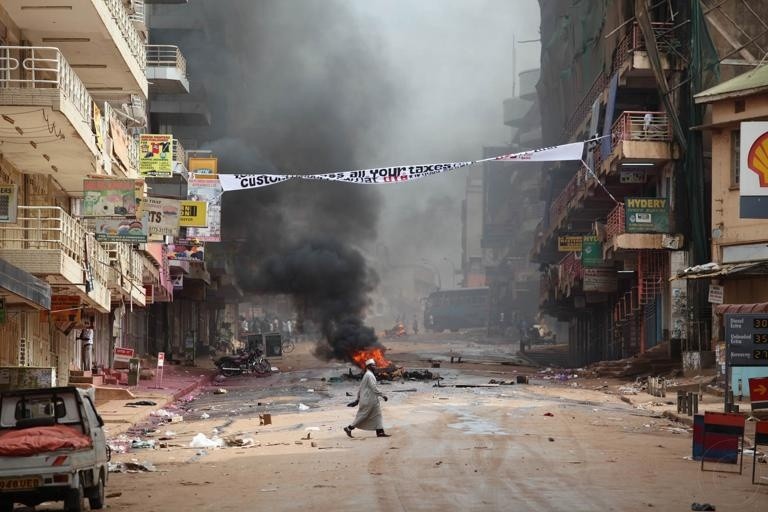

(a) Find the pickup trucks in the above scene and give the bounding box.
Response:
[2,387,110,512]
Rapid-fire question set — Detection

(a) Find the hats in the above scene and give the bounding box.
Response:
[365,358,376,366]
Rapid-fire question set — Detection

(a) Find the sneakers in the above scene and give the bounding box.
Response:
[344,426,355,438]
[376,431,391,437]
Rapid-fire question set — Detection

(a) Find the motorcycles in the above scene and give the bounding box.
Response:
[215,345,272,378]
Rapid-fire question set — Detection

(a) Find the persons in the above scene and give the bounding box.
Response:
[76,321,93,371]
[343,359,392,438]
[239,313,299,344]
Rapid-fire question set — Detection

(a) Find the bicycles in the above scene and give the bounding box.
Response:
[280,336,294,352]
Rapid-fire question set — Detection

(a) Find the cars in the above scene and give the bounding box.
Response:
[517,322,556,353]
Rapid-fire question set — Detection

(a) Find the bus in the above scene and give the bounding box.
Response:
[418,288,531,333]
